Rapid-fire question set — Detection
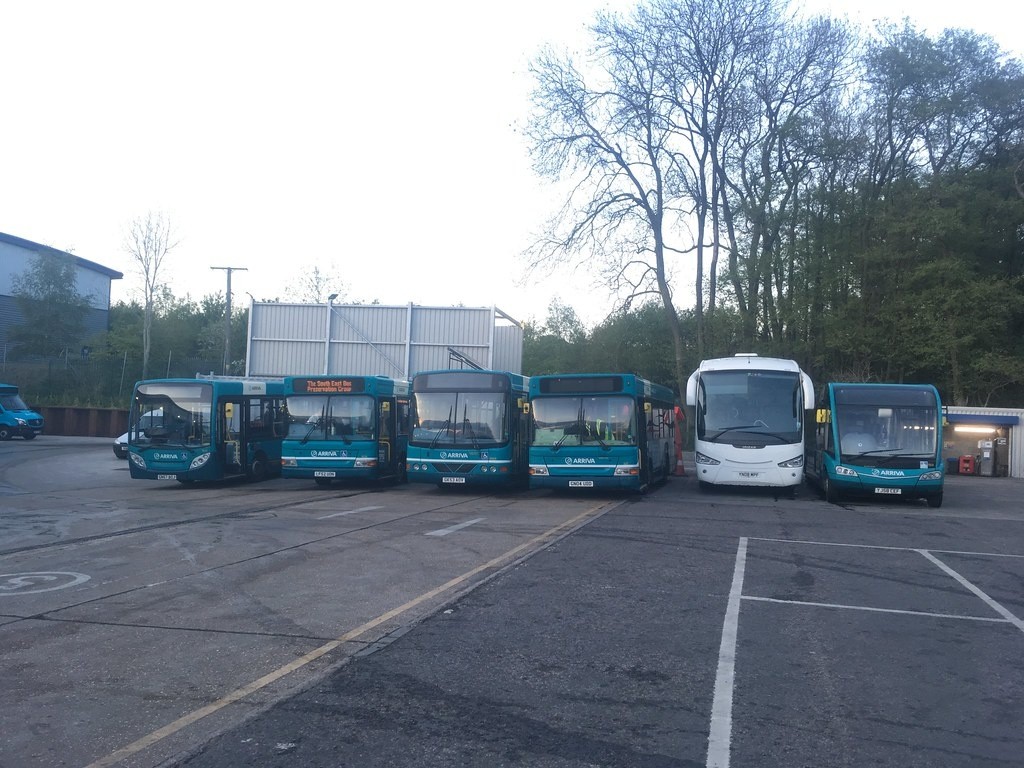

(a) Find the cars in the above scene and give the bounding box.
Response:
[112,408,164,459]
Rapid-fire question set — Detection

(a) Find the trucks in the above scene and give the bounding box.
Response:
[0,384,44,441]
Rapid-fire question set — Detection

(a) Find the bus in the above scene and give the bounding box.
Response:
[686,353,815,490]
[805,384,945,507]
[403,368,528,488]
[280,375,414,486]
[129,378,287,482]
[524,373,676,493]
